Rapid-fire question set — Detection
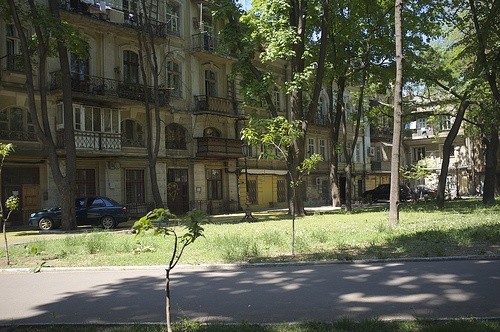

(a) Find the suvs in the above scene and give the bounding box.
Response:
[362,183,411,202]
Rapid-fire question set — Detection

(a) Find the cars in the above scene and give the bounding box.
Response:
[28,196,130,230]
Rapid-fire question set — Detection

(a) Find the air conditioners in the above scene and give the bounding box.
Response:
[316,177,323,186]
[368,147,375,157]
